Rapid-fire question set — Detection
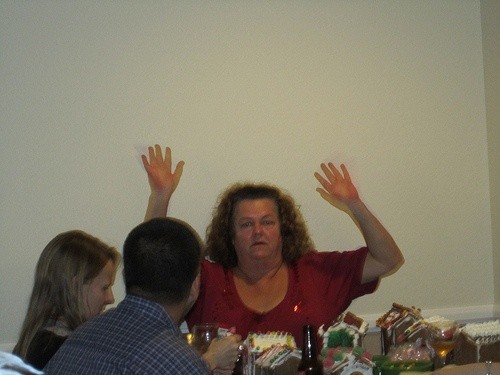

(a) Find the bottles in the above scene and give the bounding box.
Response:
[298,324,323,375]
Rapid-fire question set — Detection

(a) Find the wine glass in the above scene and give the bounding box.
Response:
[191,322,218,354]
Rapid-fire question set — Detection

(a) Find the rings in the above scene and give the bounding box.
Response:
[238,355,241,357]
[237,359,239,361]
[239,345,243,350]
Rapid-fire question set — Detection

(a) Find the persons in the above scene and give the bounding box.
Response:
[141,143,408,355]
[37,217,214,375]
[8,230,245,375]
